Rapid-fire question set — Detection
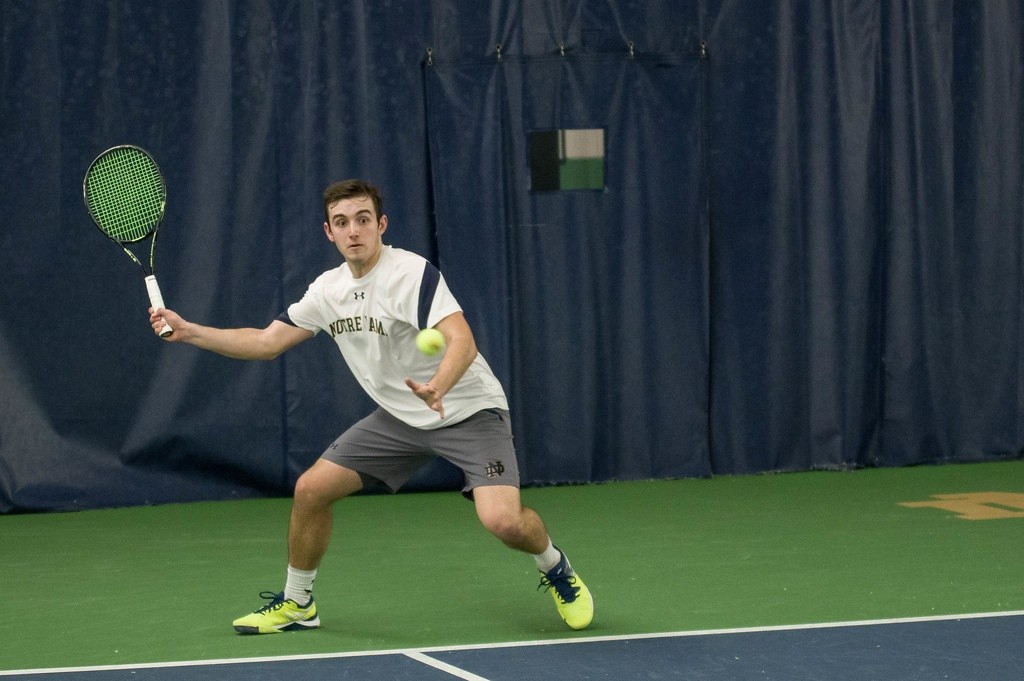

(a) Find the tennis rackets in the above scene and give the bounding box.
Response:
[83,142,174,339]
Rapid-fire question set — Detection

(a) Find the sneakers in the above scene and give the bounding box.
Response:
[538,544,594,630]
[232,590,321,634]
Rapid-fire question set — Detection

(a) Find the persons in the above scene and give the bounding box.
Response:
[149,177,594,633]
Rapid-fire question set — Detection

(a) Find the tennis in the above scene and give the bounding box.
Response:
[416,327,445,356]
